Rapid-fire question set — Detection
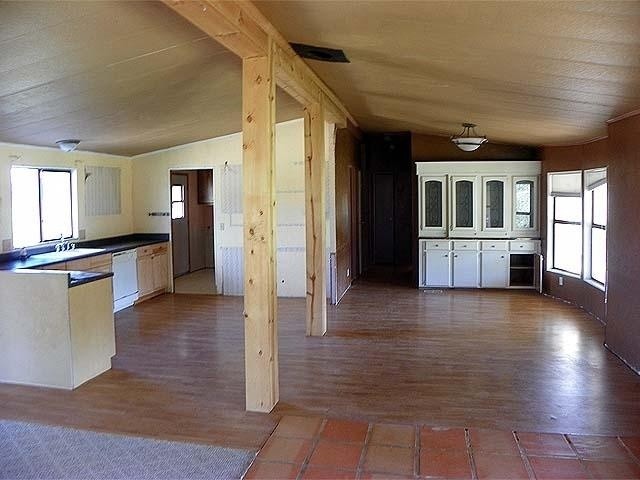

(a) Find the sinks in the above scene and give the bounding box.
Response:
[31,248,105,259]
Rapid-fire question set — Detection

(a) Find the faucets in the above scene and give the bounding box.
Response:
[55,233,70,252]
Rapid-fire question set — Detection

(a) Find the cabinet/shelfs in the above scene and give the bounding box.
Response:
[415,161,544,295]
[67,253,112,273]
[137,242,169,300]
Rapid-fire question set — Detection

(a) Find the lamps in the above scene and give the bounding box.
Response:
[449,123,489,152]
[55,139,81,153]
